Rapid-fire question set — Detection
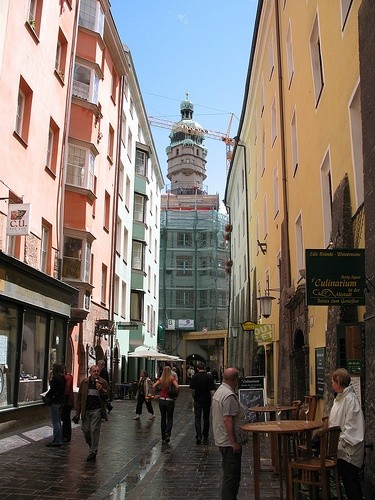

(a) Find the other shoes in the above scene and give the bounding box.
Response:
[149,416,155,420]
[166,433,170,442]
[87,454,96,461]
[46,441,63,446]
[108,406,113,414]
[133,416,140,420]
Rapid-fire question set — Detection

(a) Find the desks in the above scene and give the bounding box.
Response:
[119,383,132,400]
[251,406,298,474]
[241,420,323,500]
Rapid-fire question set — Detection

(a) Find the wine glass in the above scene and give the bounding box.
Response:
[275,404,282,424]
[303,403,309,422]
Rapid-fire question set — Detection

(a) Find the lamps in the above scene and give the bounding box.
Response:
[256,288,280,319]
[230,323,243,338]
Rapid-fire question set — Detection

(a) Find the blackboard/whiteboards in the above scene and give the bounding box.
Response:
[238,388,268,438]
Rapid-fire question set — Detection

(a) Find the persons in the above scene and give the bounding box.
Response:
[97,360,113,414]
[133,369,155,420]
[76,365,110,462]
[46,362,73,446]
[152,366,178,443]
[213,367,247,500]
[191,363,215,445]
[212,366,224,384]
[326,368,365,500]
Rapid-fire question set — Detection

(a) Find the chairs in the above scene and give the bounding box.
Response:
[287,395,343,500]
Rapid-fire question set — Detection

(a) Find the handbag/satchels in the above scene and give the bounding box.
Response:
[168,385,178,399]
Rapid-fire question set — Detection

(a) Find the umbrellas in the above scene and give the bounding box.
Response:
[127,348,186,379]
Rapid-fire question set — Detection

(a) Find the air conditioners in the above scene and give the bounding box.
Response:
[168,319,175,330]
